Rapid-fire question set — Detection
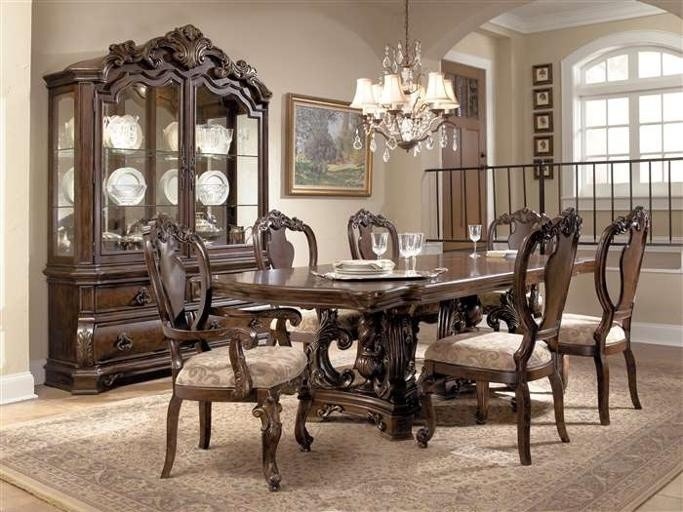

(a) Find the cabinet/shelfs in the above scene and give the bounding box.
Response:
[42,23,274,395]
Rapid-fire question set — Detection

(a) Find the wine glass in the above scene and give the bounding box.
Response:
[397,233,415,272]
[411,233,422,269]
[223,129,233,154]
[371,232,390,260]
[468,225,480,259]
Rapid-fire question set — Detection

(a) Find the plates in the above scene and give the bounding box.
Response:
[107,167,146,206]
[164,122,179,152]
[160,168,179,206]
[201,124,230,154]
[199,170,229,206]
[103,115,143,154]
[65,116,74,147]
[61,167,74,205]
[336,261,390,275]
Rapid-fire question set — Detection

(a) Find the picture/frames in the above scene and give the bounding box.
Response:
[533,112,553,133]
[281,92,373,198]
[533,159,553,180]
[533,87,553,108]
[534,135,553,156]
[532,62,552,85]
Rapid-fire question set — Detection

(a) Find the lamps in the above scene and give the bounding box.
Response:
[347,1,463,162]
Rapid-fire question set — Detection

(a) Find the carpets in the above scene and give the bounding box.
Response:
[0,343,682,512]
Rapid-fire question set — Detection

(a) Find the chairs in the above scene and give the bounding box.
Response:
[348,208,400,260]
[416,207,584,466]
[478,207,553,333]
[251,210,360,359]
[512,205,650,426]
[141,212,314,492]
